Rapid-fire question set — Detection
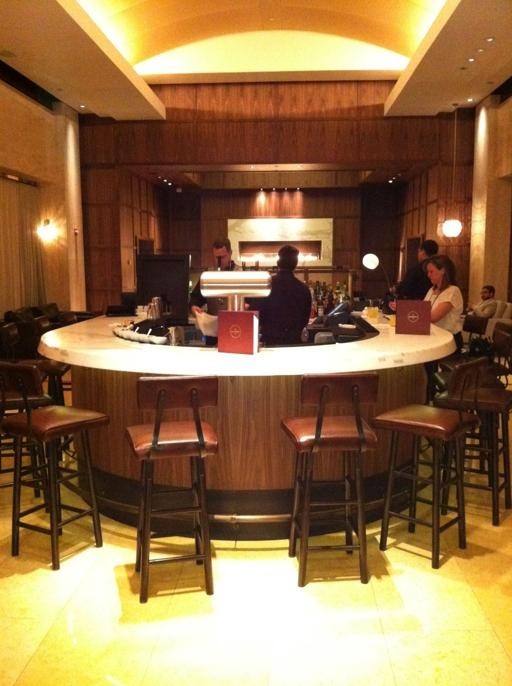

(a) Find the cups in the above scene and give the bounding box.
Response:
[365,297,382,324]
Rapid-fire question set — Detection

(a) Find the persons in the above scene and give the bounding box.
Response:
[388,254,464,376]
[189,236,243,347]
[459,283,497,318]
[242,245,312,346]
[388,239,439,301]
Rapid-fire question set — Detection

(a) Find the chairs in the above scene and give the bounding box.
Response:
[458,299,511,345]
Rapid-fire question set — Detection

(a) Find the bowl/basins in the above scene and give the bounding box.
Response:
[112,328,169,345]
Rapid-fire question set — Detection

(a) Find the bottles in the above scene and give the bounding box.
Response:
[302,280,351,316]
[136,296,162,320]
